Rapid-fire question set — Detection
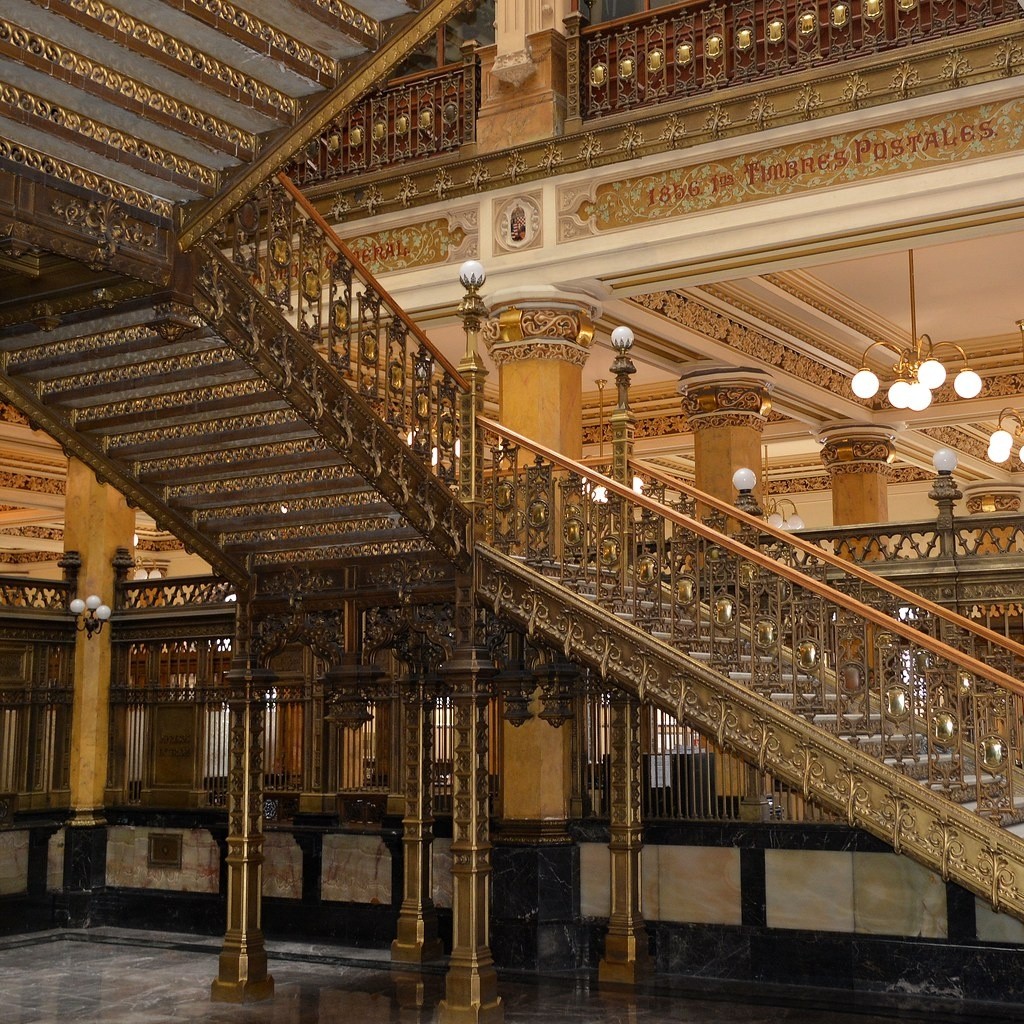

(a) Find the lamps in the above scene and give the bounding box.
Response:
[933,448,958,476]
[852,248,981,411]
[580,380,644,504]
[70,595,111,641]
[611,326,634,352]
[762,446,805,530]
[732,468,757,492]
[459,260,486,289]
[988,319,1024,464]
[135,556,161,580]
